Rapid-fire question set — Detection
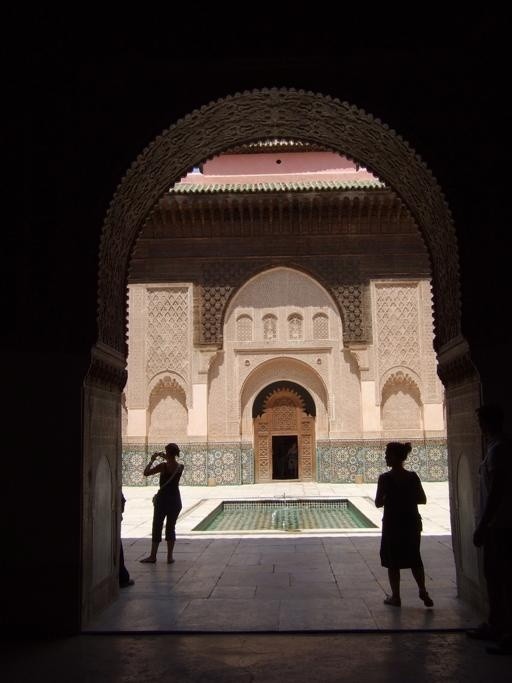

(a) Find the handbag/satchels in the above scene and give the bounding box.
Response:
[152,490,165,506]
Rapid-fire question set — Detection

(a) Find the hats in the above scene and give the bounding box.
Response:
[165,443,181,457]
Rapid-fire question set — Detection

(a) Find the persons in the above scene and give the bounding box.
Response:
[138,442,186,565]
[374,439,437,607]
[118,489,135,588]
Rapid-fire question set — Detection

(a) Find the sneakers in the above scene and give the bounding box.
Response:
[139,554,176,564]
[419,590,433,607]
[384,596,400,607]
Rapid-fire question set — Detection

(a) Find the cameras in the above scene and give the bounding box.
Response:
[158,452,165,457]
